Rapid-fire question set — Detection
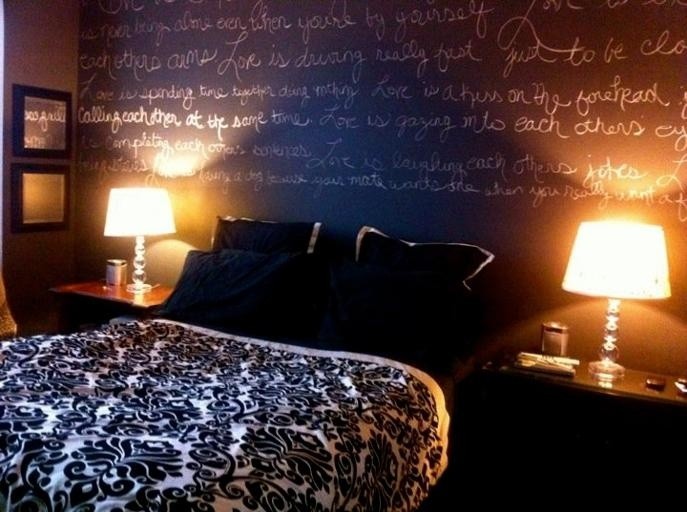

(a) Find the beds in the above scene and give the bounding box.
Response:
[1,276,496,512]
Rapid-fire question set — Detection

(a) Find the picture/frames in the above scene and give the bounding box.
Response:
[10,82,73,159]
[12,165,71,235]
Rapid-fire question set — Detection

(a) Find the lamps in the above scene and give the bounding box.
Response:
[561,220,672,383]
[104,186,176,294]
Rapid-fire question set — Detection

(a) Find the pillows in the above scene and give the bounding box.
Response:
[321,261,472,376]
[352,224,493,290]
[211,216,321,262]
[160,247,262,335]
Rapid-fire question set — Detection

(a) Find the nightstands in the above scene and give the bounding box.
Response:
[47,279,174,338]
[490,357,687,511]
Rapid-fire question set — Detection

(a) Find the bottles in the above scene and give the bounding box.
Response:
[106,259,126,285]
[541,322,569,355]
